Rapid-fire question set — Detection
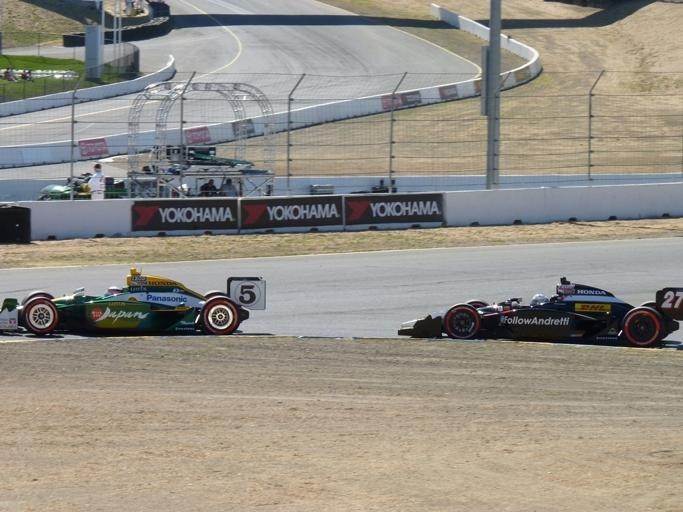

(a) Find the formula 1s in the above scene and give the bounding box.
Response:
[37,146,255,201]
[0,268,266,335]
[397,276,682,348]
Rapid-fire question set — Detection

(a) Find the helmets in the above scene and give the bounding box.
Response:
[105,287,123,296]
[529,294,550,307]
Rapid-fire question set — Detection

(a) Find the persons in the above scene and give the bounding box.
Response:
[386,179,396,193]
[3,68,13,81]
[87,164,105,200]
[20,69,28,80]
[372,179,388,193]
[9,68,17,80]
[219,178,237,197]
[27,70,32,79]
[197,179,217,197]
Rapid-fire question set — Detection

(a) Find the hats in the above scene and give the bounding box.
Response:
[94,163,102,171]
